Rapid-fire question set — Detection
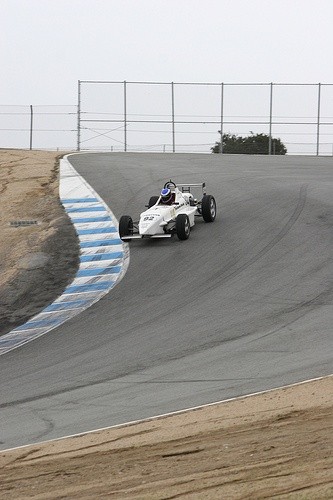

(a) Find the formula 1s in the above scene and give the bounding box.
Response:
[117,179,217,241]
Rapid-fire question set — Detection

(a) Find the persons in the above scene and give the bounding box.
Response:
[159,189,178,206]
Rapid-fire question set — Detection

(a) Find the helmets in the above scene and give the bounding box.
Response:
[160,188,172,203]
